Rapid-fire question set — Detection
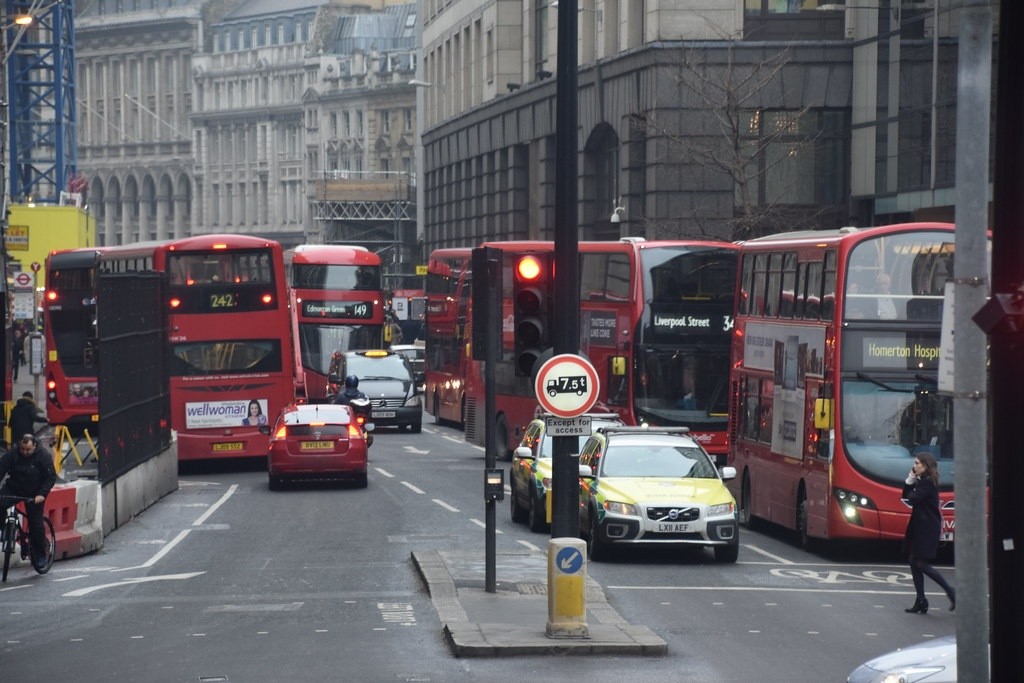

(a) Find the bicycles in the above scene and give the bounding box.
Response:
[0,495,56,582]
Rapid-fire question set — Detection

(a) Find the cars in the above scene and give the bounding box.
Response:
[390,345,426,386]
[326,348,426,433]
[579,426,741,562]
[510,414,628,532]
[258,403,375,492]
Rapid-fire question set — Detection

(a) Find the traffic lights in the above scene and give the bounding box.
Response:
[513,249,552,374]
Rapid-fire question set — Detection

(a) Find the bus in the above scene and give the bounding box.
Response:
[98,234,295,473]
[424,248,475,433]
[281,244,392,404]
[466,236,743,473]
[44,246,98,441]
[727,220,993,552]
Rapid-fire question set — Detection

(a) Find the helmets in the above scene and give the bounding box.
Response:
[345,374,359,387]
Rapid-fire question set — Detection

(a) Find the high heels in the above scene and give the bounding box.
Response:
[905,597,929,615]
[947,586,955,611]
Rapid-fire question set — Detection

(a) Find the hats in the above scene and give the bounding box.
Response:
[23,391,32,397]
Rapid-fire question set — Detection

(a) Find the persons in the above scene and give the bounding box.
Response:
[11,319,37,383]
[333,376,372,421]
[0,433,56,568]
[901,452,956,615]
[8,391,50,444]
[858,272,907,320]
[241,399,266,426]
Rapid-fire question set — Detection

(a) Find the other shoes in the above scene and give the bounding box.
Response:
[35,552,45,567]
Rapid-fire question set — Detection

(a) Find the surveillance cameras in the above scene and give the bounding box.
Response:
[611,213,621,229]
